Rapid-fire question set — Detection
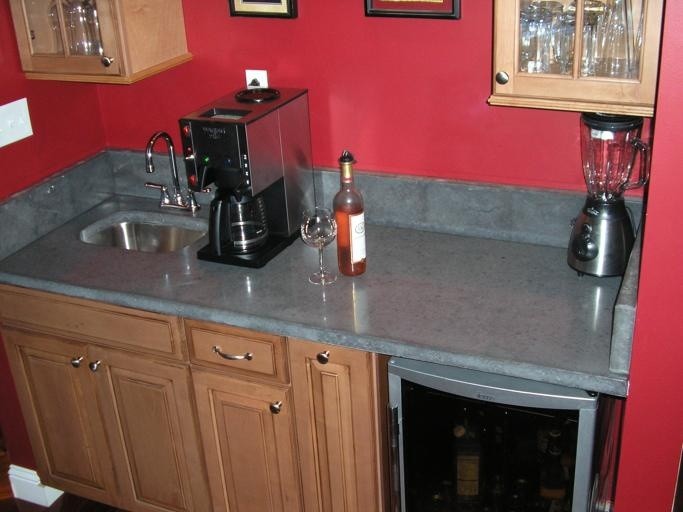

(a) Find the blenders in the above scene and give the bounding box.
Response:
[565,111,654,280]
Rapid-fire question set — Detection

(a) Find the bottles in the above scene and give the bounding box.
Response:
[452,405,484,504]
[537,426,567,502]
[332,150,367,277]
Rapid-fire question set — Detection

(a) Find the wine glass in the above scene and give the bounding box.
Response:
[299,207,338,286]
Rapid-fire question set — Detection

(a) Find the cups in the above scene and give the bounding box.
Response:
[46,0,104,56]
[517,0,645,78]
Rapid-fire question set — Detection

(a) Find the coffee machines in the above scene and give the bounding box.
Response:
[176,84,316,271]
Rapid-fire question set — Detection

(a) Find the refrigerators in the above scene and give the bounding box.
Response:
[383,355,600,511]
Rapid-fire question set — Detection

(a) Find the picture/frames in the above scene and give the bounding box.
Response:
[365,0,460,19]
[229,0,297,19]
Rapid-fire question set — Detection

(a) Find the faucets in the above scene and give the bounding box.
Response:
[145,131,187,208]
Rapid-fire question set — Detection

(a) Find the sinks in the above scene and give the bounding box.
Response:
[80,211,209,254]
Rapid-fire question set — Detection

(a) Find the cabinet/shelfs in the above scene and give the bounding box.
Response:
[487,0,666,117]
[1,280,213,511]
[182,316,305,512]
[9,0,193,85]
[285,337,385,512]
